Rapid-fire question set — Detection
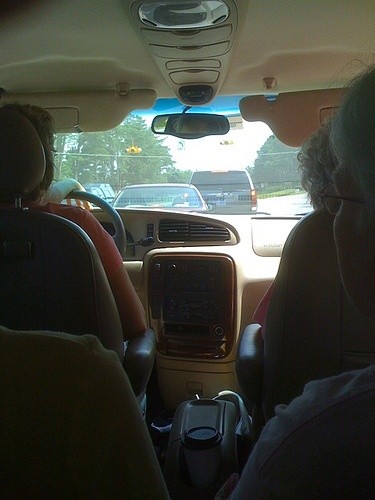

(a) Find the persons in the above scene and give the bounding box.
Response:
[1,100,147,359]
[250,111,345,343]
[214,60,374,500]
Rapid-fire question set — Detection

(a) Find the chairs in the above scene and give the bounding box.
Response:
[0,208,156,400]
[238,211,374,422]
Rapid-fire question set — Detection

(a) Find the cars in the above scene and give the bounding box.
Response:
[106,182,209,214]
[191,170,258,216]
